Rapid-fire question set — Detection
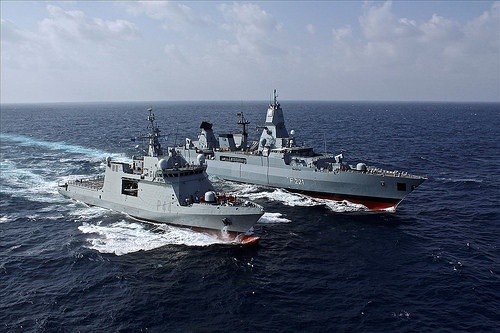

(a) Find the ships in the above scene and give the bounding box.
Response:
[55,108,265,244]
[167,90,428,212]
